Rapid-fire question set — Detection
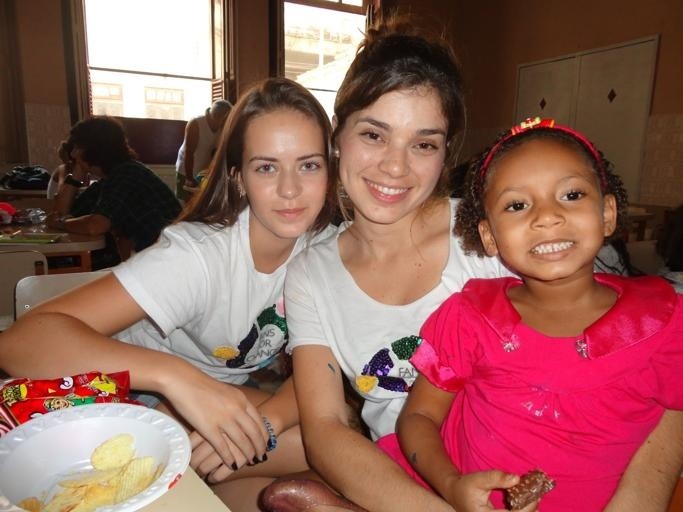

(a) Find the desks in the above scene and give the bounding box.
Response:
[0,220,107,276]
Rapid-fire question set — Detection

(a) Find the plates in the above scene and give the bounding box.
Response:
[1,402,192,512]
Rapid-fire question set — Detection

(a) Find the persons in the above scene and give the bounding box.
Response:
[255,116,682,512]
[650,205,682,296]
[285,29,502,511]
[45,141,90,201]
[172,98,233,205]
[43,114,184,267]
[0,78,346,511]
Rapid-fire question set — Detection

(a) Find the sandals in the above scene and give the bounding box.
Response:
[257,479,366,511]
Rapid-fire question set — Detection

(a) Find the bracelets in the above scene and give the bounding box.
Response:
[64,173,83,188]
[255,410,276,456]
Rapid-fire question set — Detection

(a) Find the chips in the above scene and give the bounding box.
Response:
[19,430,166,512]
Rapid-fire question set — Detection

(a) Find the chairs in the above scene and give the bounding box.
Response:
[0,250,119,333]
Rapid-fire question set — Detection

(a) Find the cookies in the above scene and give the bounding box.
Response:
[507,468,556,510]
